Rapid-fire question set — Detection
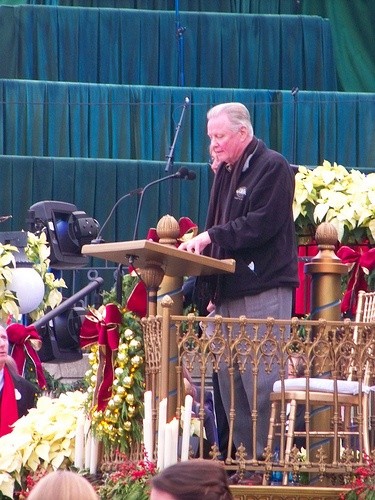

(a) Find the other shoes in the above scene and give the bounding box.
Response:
[226,474,262,485]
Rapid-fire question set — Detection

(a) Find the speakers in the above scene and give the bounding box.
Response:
[27,296,83,363]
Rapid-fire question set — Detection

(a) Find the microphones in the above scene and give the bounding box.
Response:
[133,166,197,240]
[91,187,144,244]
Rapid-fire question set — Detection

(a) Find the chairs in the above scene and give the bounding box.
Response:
[262,290,375,486]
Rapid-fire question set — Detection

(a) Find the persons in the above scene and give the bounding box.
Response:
[0,325,35,438]
[148,461,234,500]
[201,144,237,476]
[176,101,300,487]
